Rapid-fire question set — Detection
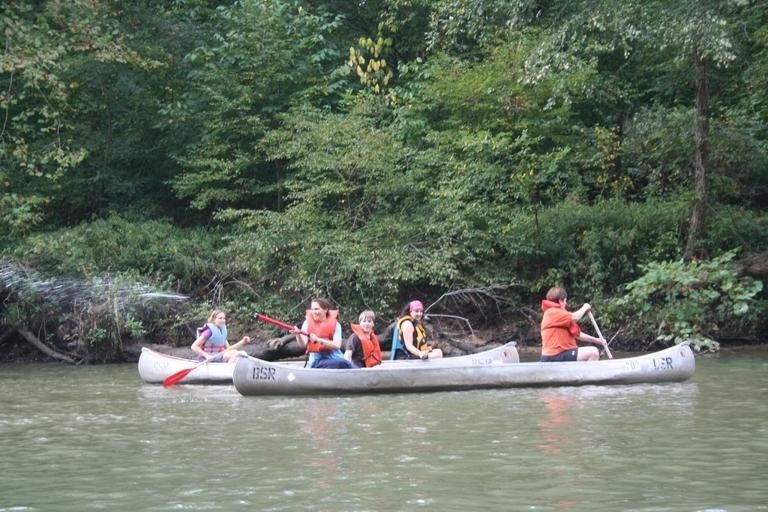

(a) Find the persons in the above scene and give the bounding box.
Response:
[190,310,251,364]
[539,287,607,362]
[393,301,439,361]
[288,299,358,369]
[343,310,381,369]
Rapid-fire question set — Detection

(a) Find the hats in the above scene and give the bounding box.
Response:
[410,300,424,311]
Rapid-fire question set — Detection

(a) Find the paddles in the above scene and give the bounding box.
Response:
[164,340,243,387]
